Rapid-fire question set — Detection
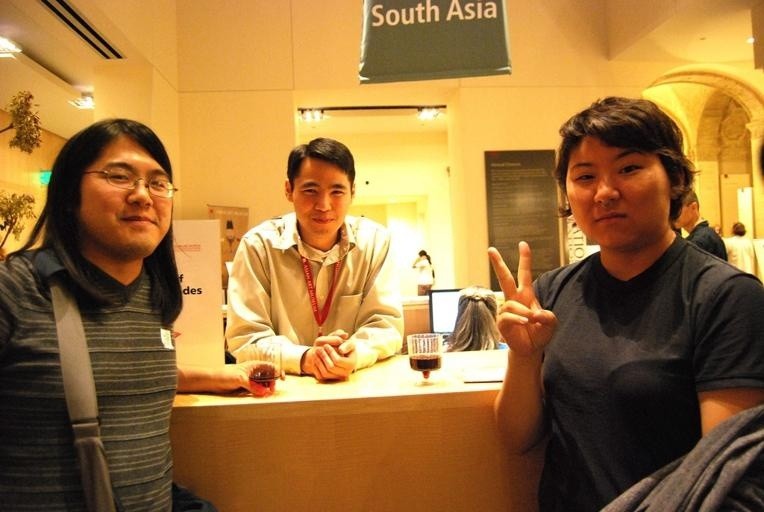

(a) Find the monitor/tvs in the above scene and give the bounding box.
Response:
[429,289,462,335]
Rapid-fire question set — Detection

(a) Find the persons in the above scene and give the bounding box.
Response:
[413,248,436,297]
[720,221,757,282]
[439,286,514,353]
[486,95,763,511]
[225,135,406,384]
[663,187,728,266]
[0,117,286,512]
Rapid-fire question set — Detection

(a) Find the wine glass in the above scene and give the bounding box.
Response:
[240,346,283,397]
[406,334,443,388]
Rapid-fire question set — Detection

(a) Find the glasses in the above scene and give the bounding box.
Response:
[84,169,178,199]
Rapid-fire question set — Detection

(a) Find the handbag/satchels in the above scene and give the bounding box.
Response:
[49,282,219,512]
